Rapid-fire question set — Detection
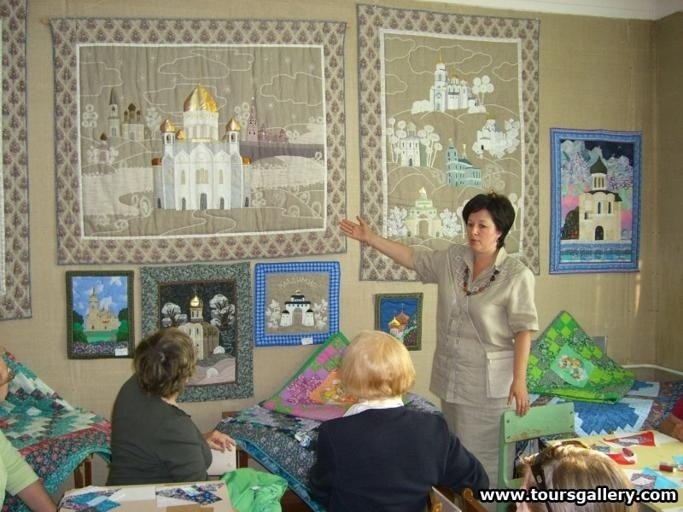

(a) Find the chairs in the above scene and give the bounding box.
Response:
[495,402,577,511]
[461,487,489,511]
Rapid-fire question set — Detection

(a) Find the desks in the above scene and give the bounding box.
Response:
[2,437,94,493]
[545,429,683,512]
[218,411,445,512]
[57,465,290,512]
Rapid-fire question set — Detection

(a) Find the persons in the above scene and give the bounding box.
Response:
[0,360,59,512]
[518,436,633,512]
[305,329,491,512]
[339,192,539,512]
[104,325,238,486]
